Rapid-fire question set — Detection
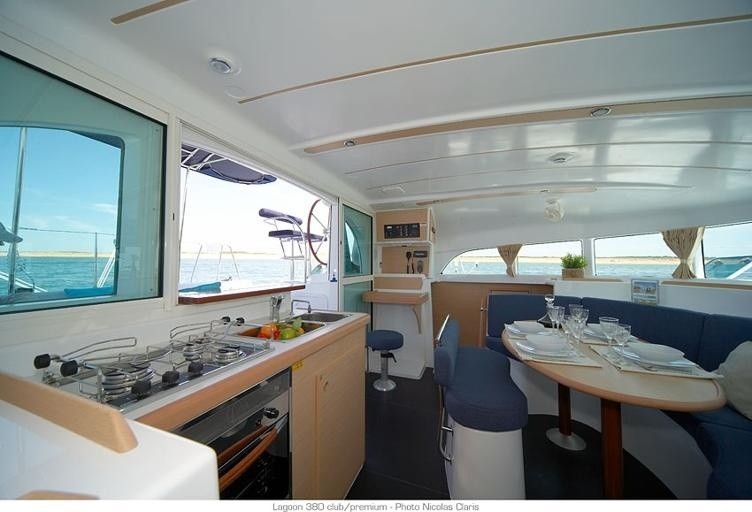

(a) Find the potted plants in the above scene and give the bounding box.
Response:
[561,254,587,282]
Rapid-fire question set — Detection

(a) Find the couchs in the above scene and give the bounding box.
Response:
[487,288,752,503]
[434,312,524,502]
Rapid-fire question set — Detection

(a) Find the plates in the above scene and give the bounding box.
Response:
[507,325,553,337]
[612,345,696,371]
[584,326,608,338]
[516,341,577,358]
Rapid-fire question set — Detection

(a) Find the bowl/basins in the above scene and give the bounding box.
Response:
[525,334,567,351]
[514,321,544,333]
[587,323,602,334]
[626,341,684,363]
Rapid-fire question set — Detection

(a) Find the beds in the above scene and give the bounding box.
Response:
[503,312,728,502]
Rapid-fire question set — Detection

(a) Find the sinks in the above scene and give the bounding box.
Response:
[237,321,325,341]
[291,311,354,322]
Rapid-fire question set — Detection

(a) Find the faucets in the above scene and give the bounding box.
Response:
[269,294,285,321]
[289,299,312,315]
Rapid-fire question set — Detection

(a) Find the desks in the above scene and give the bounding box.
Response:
[363,291,428,334]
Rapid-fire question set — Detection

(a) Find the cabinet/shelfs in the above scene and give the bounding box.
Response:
[292,325,365,500]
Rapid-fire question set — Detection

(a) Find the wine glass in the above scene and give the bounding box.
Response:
[572,307,590,346]
[568,304,583,315]
[559,315,574,338]
[611,323,632,364]
[545,305,558,337]
[599,317,619,357]
[556,306,565,336]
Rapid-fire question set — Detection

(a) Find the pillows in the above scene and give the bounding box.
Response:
[706,341,752,416]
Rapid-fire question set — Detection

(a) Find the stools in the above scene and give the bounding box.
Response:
[369,326,405,394]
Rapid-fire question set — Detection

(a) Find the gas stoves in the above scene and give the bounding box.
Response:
[33,315,275,415]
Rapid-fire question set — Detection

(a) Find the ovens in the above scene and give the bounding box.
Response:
[170,366,292,500]
